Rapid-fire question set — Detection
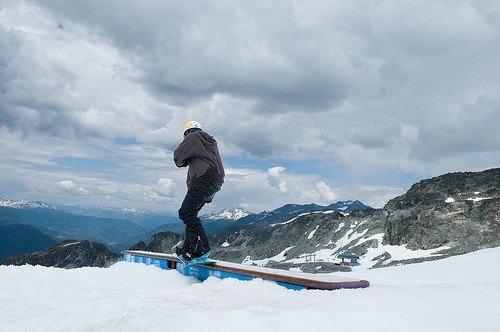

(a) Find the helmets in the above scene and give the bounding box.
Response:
[183,121,203,135]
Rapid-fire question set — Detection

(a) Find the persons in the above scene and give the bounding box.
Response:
[174,121,225,260]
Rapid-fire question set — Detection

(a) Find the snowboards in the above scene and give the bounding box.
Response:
[174,245,217,267]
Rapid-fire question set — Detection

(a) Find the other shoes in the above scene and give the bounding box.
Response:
[190,249,212,259]
[176,246,191,259]
